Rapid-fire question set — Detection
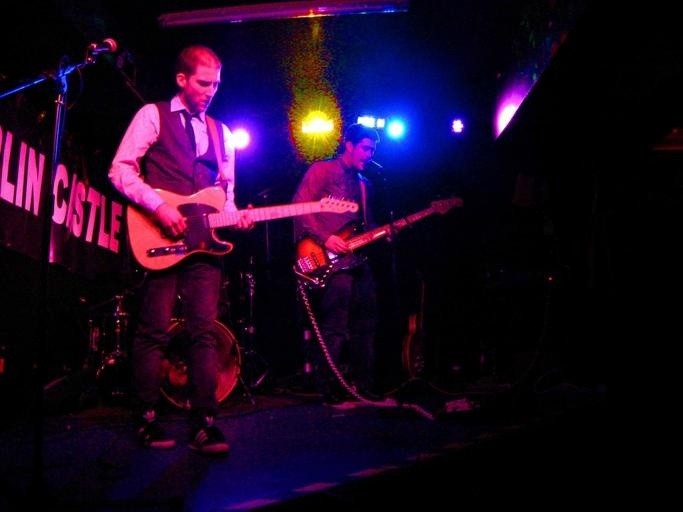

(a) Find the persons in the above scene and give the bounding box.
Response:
[109,47,255,452]
[293,124,395,404]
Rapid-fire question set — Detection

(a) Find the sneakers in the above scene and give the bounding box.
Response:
[133,422,176,450]
[183,423,230,457]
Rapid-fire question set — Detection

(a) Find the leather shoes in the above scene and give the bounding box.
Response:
[322,387,342,403]
[359,380,385,402]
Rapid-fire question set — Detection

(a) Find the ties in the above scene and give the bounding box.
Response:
[182,110,196,154]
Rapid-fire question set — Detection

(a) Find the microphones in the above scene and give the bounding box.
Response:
[103,294,120,304]
[85,38,118,64]
[368,159,383,169]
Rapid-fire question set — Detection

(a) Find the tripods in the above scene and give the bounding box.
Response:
[84,308,134,403]
[2,63,86,470]
[242,271,277,399]
[377,176,484,410]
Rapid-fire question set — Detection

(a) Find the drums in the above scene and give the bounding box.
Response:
[159,318,242,410]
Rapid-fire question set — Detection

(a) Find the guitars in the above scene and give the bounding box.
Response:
[402,281,427,377]
[297,196,464,289]
[126,184,359,271]
